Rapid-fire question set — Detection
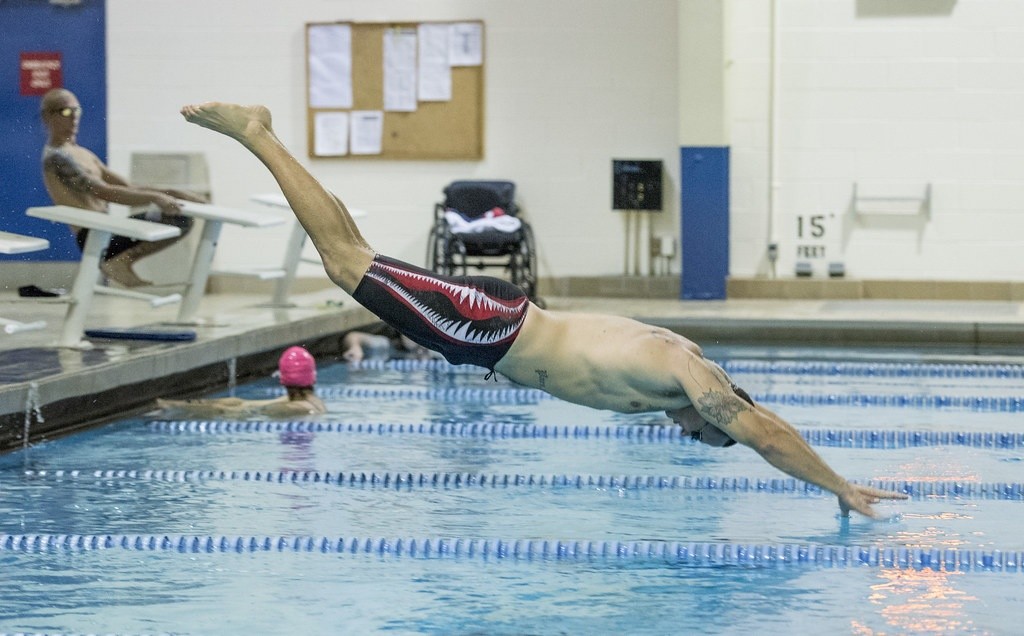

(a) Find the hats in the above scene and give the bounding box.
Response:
[279,346,315,387]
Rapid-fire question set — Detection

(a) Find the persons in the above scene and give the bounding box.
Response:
[41,90,205,289]
[153,346,326,421]
[344,325,447,364]
[179,104,909,522]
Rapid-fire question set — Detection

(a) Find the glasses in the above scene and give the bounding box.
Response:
[690,430,702,441]
[60,107,82,118]
